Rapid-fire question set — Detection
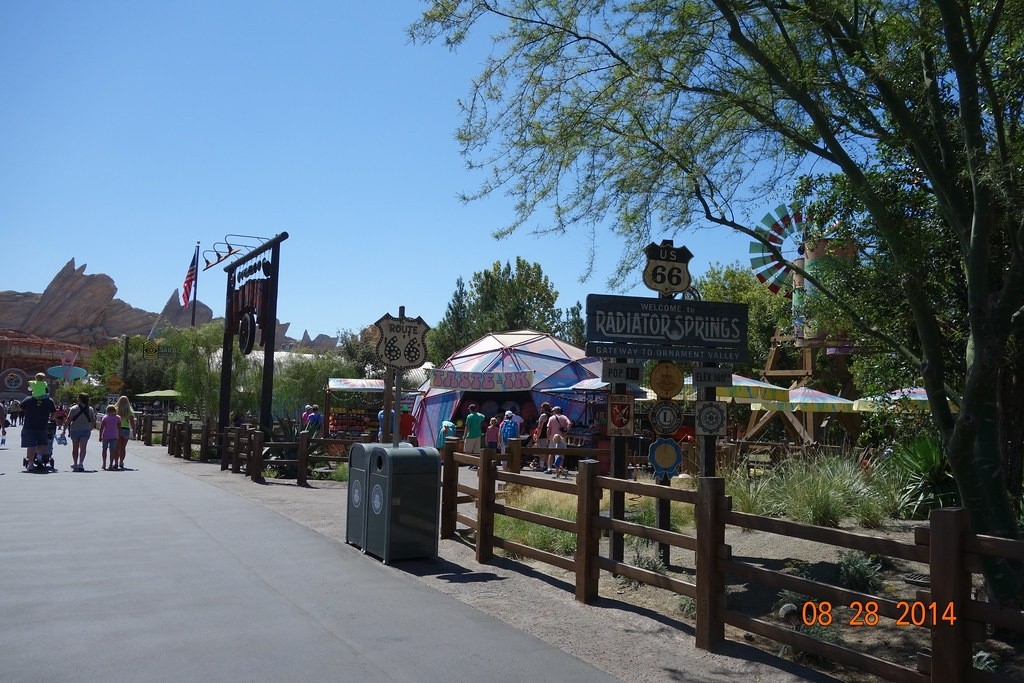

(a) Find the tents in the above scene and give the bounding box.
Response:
[136,390,183,399]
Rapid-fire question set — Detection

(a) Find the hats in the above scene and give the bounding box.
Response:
[305,405,312,409]
[400,404,411,411]
[505,411,514,417]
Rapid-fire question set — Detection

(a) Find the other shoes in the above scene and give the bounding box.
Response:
[552,474,559,479]
[70,464,83,471]
[14,424,16,427]
[11,423,14,427]
[102,463,124,471]
[27,463,34,471]
[544,470,552,474]
[37,465,44,471]
[470,466,478,470]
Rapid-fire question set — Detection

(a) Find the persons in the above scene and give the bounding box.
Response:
[1,396,76,436]
[461,402,574,474]
[378,402,418,444]
[230,403,247,427]
[437,420,457,463]
[98,396,136,472]
[61,393,96,472]
[18,372,56,471]
[552,434,569,479]
[302,404,323,435]
[0,398,7,437]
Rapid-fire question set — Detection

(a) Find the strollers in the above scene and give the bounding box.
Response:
[23,419,56,468]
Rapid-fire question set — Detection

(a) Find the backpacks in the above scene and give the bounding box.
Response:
[310,415,320,430]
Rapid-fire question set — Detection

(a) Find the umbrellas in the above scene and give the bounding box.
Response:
[632,369,965,445]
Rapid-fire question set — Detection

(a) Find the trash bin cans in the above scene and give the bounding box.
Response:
[346,443,441,564]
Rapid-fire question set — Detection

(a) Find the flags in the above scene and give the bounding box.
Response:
[182,245,198,310]
